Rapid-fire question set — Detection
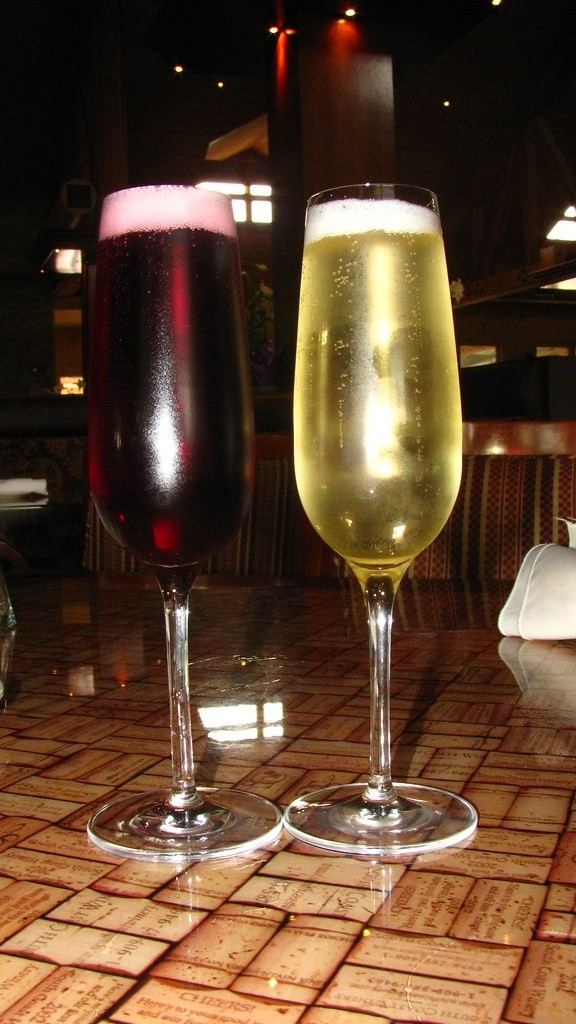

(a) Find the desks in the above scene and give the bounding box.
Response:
[0,581,576,1023]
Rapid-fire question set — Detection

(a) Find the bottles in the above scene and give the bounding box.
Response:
[0,569,18,710]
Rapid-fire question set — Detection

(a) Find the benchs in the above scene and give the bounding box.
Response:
[68,420,575,579]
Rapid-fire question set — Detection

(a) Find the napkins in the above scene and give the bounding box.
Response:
[496,518,576,641]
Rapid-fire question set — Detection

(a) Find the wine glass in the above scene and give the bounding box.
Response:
[281,179,479,863]
[85,184,286,868]
